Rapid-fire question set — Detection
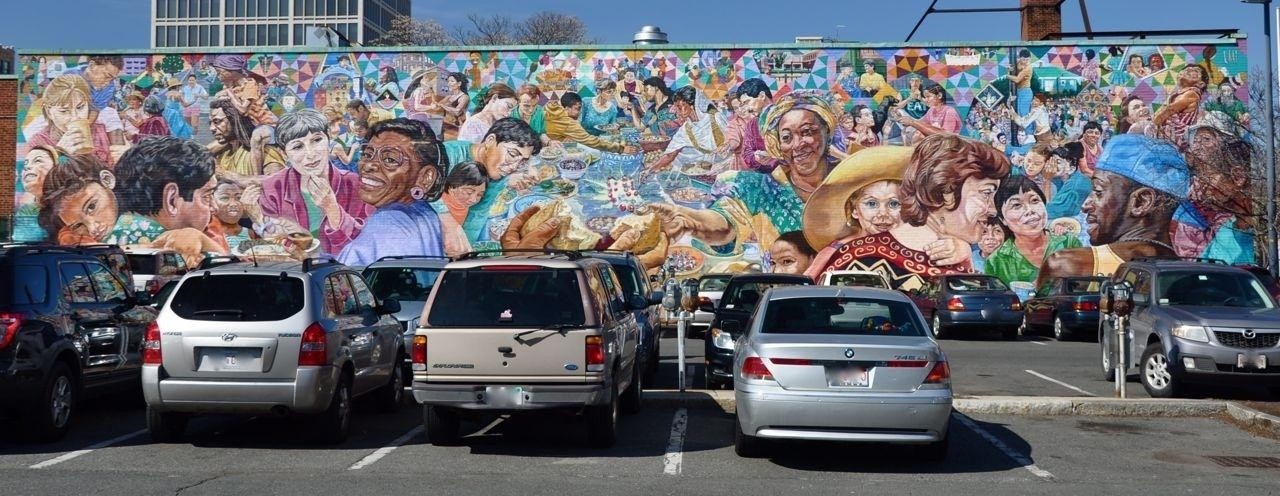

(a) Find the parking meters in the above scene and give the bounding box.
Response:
[1112,279,1135,398]
[1099,277,1119,396]
[661,278,700,393]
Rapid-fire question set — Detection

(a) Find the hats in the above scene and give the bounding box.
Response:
[803,142,919,254]
[1096,133,1190,201]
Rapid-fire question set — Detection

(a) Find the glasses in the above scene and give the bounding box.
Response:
[356,141,424,171]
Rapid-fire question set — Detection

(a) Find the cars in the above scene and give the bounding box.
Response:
[817,269,892,290]
[141,242,407,444]
[360,253,454,386]
[1097,260,1280,395]
[412,246,644,447]
[931,273,1025,339]
[0,241,159,445]
[118,243,190,297]
[686,272,734,340]
[705,271,817,380]
[1019,275,1134,342]
[575,247,663,414]
[81,241,136,296]
[734,286,954,463]
